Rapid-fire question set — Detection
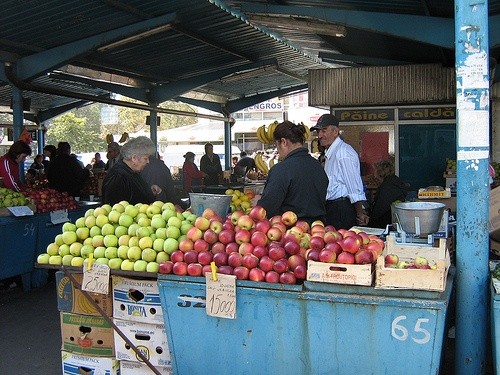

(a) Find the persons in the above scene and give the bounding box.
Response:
[309,113,370,230]
[234,151,258,173]
[105,142,122,170]
[182,153,205,198]
[26,168,36,179]
[200,142,222,186]
[232,157,238,167]
[93,152,105,170]
[0,140,32,191]
[317,140,325,169]
[101,138,162,206]
[138,136,174,202]
[252,120,329,225]
[360,161,372,213]
[85,158,95,169]
[30,154,43,169]
[370,160,407,228]
[42,142,92,201]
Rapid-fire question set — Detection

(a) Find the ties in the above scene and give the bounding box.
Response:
[113,159,115,165]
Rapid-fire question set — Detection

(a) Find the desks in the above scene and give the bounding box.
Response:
[206,181,265,194]
[0,201,102,291]
[155,272,453,375]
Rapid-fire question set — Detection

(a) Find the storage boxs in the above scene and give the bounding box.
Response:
[55,271,172,375]
[306,260,376,286]
[373,235,450,292]
[418,177,457,199]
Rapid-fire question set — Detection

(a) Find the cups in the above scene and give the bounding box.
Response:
[75,197,80,201]
[89,195,95,201]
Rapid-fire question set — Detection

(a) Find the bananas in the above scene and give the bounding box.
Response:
[119,132,126,143]
[106,134,113,144]
[257,121,311,143]
[255,152,282,173]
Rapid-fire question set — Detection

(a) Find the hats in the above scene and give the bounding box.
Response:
[310,114,339,132]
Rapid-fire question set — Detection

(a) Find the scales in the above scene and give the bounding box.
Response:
[388,201,448,247]
[189,192,233,222]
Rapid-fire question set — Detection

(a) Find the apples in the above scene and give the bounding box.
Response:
[27,173,97,195]
[0,188,30,208]
[20,188,79,212]
[447,160,456,170]
[37,201,198,273]
[224,189,262,214]
[159,206,385,284]
[384,253,444,269]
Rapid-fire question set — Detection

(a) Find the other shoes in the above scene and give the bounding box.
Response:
[8,282,18,291]
